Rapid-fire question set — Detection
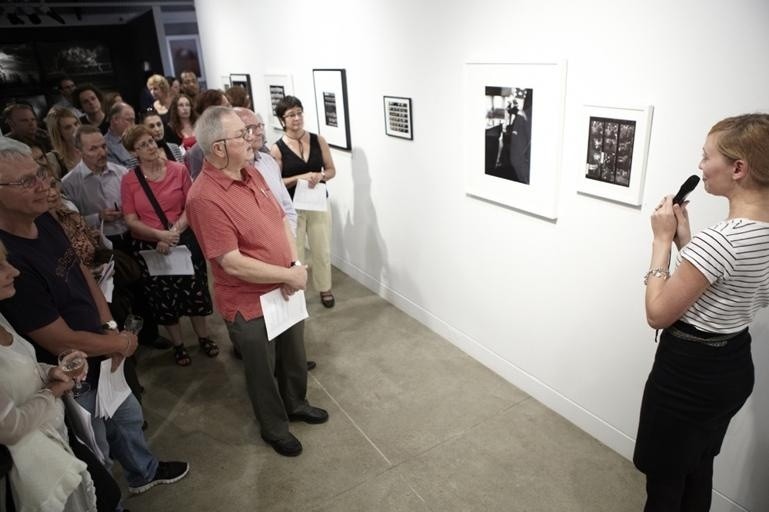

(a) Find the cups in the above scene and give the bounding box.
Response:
[123,313,145,333]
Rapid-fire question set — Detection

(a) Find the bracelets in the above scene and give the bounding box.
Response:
[121,337,131,354]
[644,268,670,286]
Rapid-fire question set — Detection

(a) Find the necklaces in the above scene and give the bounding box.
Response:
[286,131,306,159]
[144,163,162,180]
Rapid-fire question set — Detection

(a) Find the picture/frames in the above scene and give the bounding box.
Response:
[576,103,654,207]
[466,59,567,221]
[313,69,351,151]
[382,96,413,140]
[165,34,206,83]
[263,74,294,131]
[230,74,254,113]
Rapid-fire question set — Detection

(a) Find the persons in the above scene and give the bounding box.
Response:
[1,149,191,511]
[185,89,233,183]
[226,87,251,109]
[632,113,768,511]
[2,73,219,366]
[1,244,96,511]
[270,96,337,309]
[185,105,329,458]
[236,107,316,376]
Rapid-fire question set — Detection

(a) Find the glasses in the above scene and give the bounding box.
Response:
[211,129,252,149]
[1,164,48,190]
[246,123,265,132]
[282,109,304,119]
[133,138,156,152]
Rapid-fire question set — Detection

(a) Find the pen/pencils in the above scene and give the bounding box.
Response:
[114,202,118,211]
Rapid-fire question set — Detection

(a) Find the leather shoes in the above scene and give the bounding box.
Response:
[152,335,171,350]
[286,404,329,424]
[306,359,317,372]
[260,429,303,457]
[320,290,335,309]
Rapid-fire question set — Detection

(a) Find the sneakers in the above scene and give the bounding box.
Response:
[126,460,191,496]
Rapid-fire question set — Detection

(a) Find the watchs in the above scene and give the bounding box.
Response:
[321,172,325,179]
[290,261,300,266]
[102,320,118,331]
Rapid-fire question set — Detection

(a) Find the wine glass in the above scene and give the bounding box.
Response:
[57,346,92,398]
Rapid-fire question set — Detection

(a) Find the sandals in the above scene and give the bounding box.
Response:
[197,334,219,358]
[173,341,192,367]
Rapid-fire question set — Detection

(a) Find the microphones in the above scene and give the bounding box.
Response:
[673,175,701,207]
